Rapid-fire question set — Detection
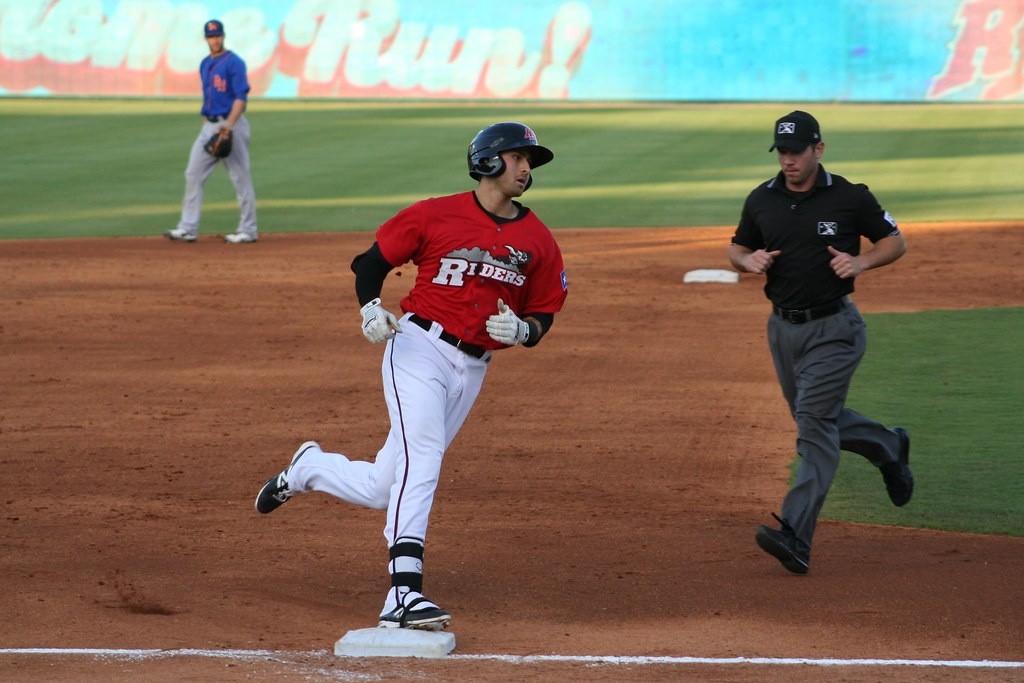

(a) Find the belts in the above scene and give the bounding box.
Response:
[206,113,228,122]
[408,315,490,362]
[773,297,844,324]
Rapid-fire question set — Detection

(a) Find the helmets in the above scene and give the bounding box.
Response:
[468,122,554,192]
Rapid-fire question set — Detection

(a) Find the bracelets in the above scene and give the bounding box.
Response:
[522,320,538,346]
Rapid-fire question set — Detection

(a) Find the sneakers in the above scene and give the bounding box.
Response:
[754,511,809,574]
[879,427,914,506]
[379,591,451,630]
[254,441,319,514]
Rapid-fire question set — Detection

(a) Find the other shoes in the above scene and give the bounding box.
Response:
[224,233,256,243]
[162,229,197,242]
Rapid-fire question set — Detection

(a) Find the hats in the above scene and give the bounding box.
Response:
[769,111,822,153]
[204,19,223,37]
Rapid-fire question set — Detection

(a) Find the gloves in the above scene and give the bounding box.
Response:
[359,298,403,343]
[486,298,529,344]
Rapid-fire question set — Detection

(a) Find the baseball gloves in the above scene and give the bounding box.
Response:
[204,126,237,159]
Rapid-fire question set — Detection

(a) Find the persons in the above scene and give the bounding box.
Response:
[728,110,914,574]
[254,123,569,630]
[162,21,259,243]
